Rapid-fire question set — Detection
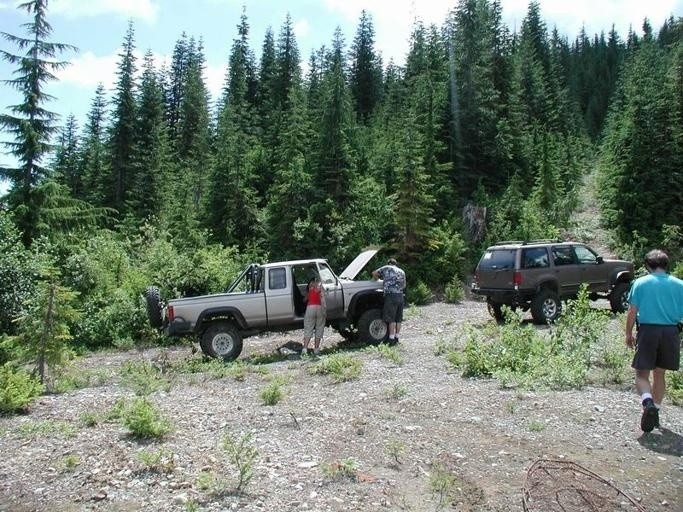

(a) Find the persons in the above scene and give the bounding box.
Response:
[625,249,683,432]
[302,277,326,355]
[372,258,406,346]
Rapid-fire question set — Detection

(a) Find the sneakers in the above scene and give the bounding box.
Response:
[387,338,398,345]
[302,347,321,354]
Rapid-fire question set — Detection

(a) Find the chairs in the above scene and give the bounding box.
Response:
[292,269,306,315]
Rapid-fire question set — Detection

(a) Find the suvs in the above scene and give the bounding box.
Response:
[471,239,634,324]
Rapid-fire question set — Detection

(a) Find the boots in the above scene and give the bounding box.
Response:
[640,399,659,433]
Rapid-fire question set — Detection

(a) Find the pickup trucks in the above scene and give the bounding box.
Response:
[148,249,390,362]
